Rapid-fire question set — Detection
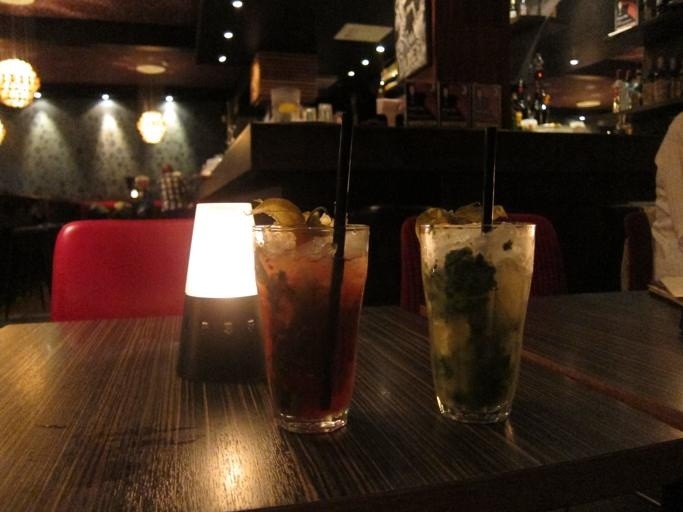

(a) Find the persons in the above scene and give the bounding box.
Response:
[440,84,468,122]
[474,86,498,123]
[154,162,189,217]
[130,175,154,219]
[405,81,438,121]
[652,111,682,284]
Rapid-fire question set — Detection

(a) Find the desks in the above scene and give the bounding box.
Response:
[3,288,681,510]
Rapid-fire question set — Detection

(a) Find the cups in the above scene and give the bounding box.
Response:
[250,225,367,438]
[415,217,537,424]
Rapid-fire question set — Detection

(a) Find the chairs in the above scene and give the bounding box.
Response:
[395,217,559,301]
[52,217,256,322]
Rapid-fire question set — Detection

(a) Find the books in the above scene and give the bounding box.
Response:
[647,275,683,309]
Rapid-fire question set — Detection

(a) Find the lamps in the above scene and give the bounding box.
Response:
[176,197,263,379]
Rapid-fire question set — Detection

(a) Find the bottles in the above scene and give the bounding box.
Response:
[612,56,683,111]
[512,70,550,129]
